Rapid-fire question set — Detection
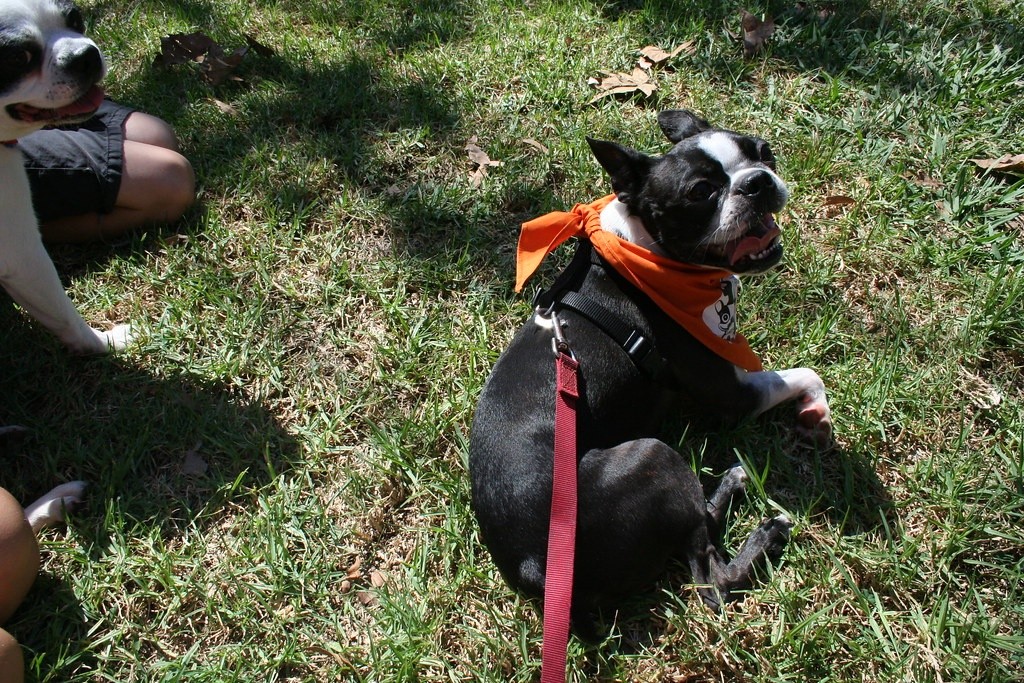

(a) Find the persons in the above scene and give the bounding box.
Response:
[0,486,39,683]
[15,100,195,243]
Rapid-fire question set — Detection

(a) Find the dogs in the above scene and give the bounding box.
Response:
[468,110,834,651]
[0,1,150,533]
